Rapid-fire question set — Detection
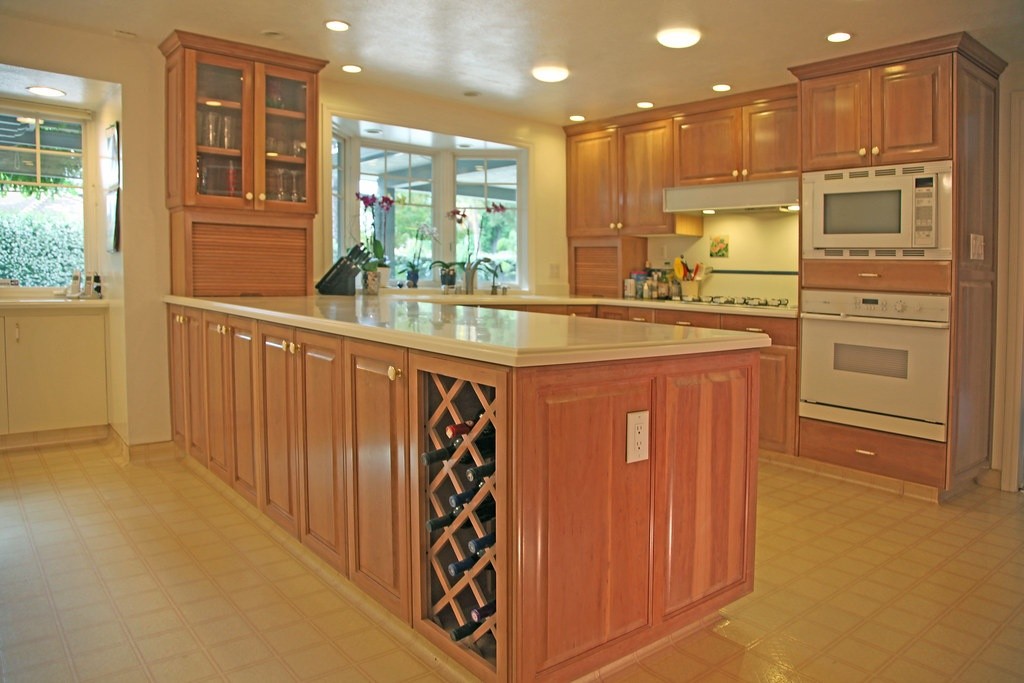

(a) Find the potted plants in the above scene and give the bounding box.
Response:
[429,260,465,288]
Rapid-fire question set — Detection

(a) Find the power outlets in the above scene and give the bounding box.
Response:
[627,410,648,463]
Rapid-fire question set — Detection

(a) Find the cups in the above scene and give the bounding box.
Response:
[224,158,239,196]
[361,271,380,296]
[267,134,304,158]
[680,281,698,301]
[196,110,235,148]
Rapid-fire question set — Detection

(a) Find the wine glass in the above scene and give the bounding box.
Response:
[420,408,496,640]
[269,167,303,202]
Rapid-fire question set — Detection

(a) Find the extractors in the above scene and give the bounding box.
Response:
[662,176,800,214]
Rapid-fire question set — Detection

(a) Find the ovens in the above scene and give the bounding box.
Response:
[797,287,951,444]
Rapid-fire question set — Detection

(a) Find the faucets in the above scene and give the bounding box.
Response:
[463,251,491,295]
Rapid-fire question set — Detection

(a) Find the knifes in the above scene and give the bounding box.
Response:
[345,242,370,269]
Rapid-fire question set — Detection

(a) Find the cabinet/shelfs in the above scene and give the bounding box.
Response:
[0,308,110,449]
[164,47,319,215]
[461,53,1001,490]
[165,301,761,683]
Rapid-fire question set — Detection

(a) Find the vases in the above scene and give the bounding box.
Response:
[377,267,392,288]
[407,271,419,288]
[465,270,474,294]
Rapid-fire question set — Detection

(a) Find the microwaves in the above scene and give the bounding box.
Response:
[801,160,953,261]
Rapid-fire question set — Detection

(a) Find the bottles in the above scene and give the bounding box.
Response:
[623,260,680,300]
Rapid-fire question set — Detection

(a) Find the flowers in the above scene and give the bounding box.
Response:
[355,190,508,278]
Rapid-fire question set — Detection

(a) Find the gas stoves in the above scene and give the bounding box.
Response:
[690,294,798,311]
